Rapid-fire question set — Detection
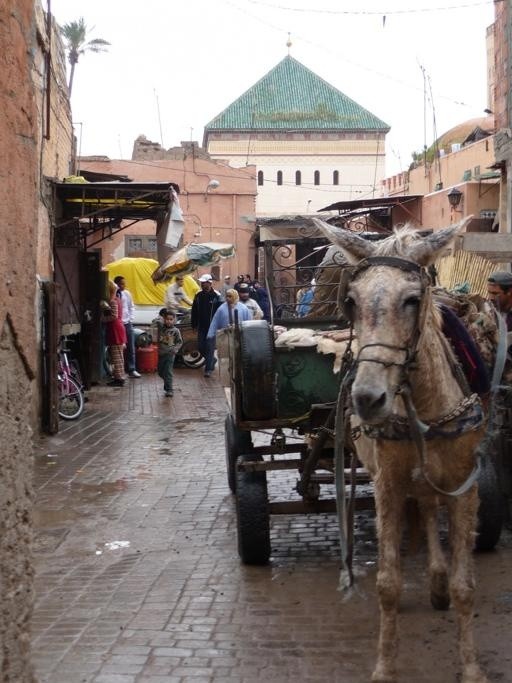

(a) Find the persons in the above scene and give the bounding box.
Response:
[101,280,128,386]
[205,288,251,338]
[191,274,222,377]
[152,307,168,326]
[485,269,511,357]
[110,276,141,378]
[165,273,317,319]
[155,309,183,397]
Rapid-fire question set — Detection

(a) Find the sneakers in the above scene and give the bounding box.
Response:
[165,392,173,396]
[106,379,125,386]
[129,370,142,377]
[204,370,212,377]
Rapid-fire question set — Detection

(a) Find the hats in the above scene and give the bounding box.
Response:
[197,274,213,282]
[488,271,512,285]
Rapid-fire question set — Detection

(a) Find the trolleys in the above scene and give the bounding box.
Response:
[173,315,209,370]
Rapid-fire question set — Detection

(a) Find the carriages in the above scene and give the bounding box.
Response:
[213,213,506,683]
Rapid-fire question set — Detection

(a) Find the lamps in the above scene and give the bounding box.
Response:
[205,178,220,202]
[448,187,464,212]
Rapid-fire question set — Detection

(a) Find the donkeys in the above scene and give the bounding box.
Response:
[311,214,492,683]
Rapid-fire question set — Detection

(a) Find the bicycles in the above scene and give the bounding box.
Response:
[56,334,89,421]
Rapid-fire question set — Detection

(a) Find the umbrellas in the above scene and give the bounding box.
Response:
[151,240,235,285]
